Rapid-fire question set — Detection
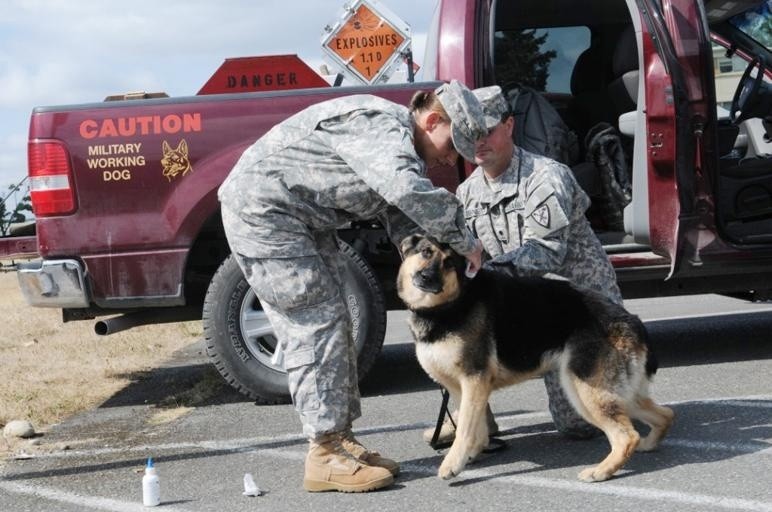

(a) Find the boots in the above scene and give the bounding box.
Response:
[335,429,400,478]
[423,403,500,447]
[304,429,395,495]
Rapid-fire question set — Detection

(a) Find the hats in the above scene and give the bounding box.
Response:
[434,77,487,166]
[470,83,509,130]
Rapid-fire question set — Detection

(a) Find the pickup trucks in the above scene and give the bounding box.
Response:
[1,0,772,405]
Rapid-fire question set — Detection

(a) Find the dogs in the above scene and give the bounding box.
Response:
[397,233,674,483]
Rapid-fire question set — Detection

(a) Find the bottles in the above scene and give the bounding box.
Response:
[142,455,161,507]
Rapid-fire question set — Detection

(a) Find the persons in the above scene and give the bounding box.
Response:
[424,85,628,443]
[220,78,490,493]
